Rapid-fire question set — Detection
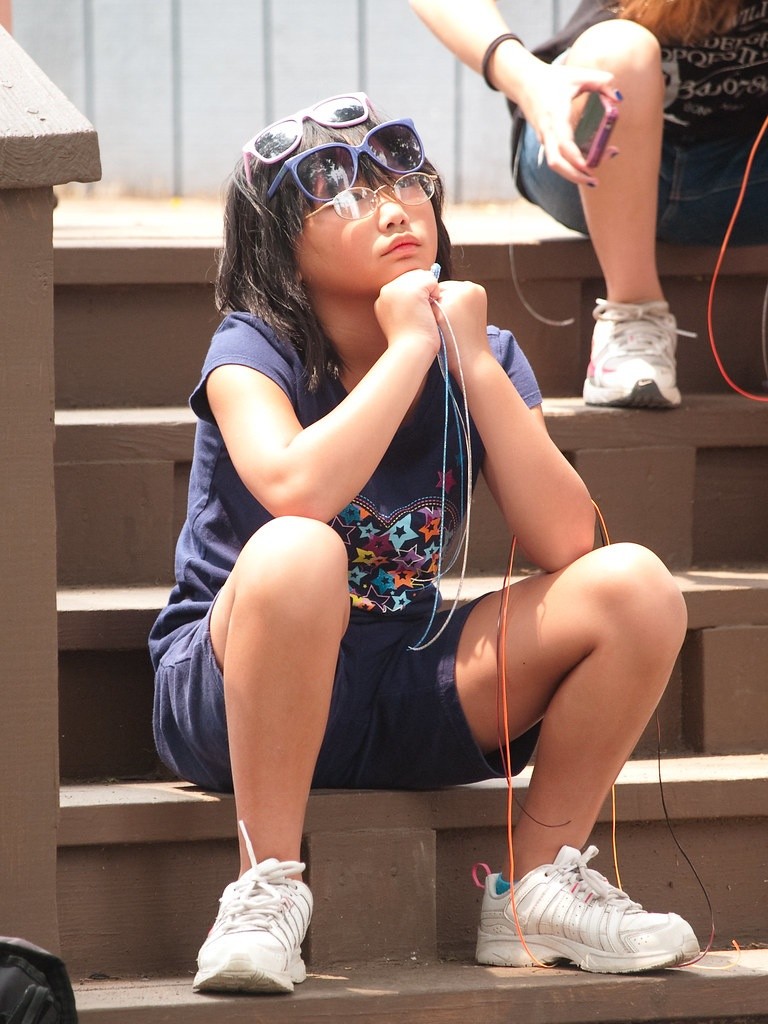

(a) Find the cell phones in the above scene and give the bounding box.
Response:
[572,92,617,168]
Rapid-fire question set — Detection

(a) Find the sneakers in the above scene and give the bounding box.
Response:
[585,306,682,408]
[475,847,696,973]
[190,878,315,996]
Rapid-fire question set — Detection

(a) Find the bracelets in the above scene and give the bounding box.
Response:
[481,33,526,92]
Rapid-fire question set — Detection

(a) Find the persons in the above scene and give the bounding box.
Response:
[148,104,701,992]
[404,0,768,410]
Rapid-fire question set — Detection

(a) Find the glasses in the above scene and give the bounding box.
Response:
[268,119,424,198]
[242,91,376,184]
[303,171,437,223]
